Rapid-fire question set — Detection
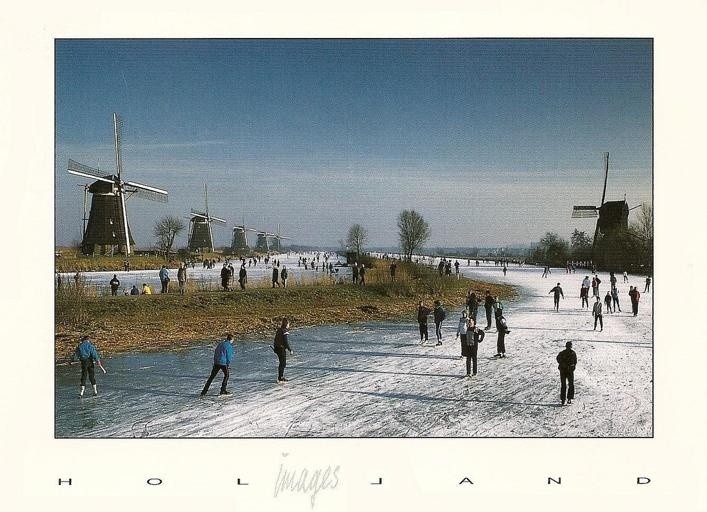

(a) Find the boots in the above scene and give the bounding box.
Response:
[92,384,98,396]
[79,386,86,396]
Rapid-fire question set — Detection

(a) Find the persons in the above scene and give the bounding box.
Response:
[273,318,293,384]
[200,334,235,397]
[297,242,521,285]
[417,302,430,345]
[454,289,507,376]
[75,336,100,396]
[433,300,444,346]
[556,341,577,404]
[110,252,287,297]
[542,254,650,331]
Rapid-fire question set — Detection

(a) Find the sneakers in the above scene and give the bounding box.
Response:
[220,391,232,395]
[277,377,288,381]
